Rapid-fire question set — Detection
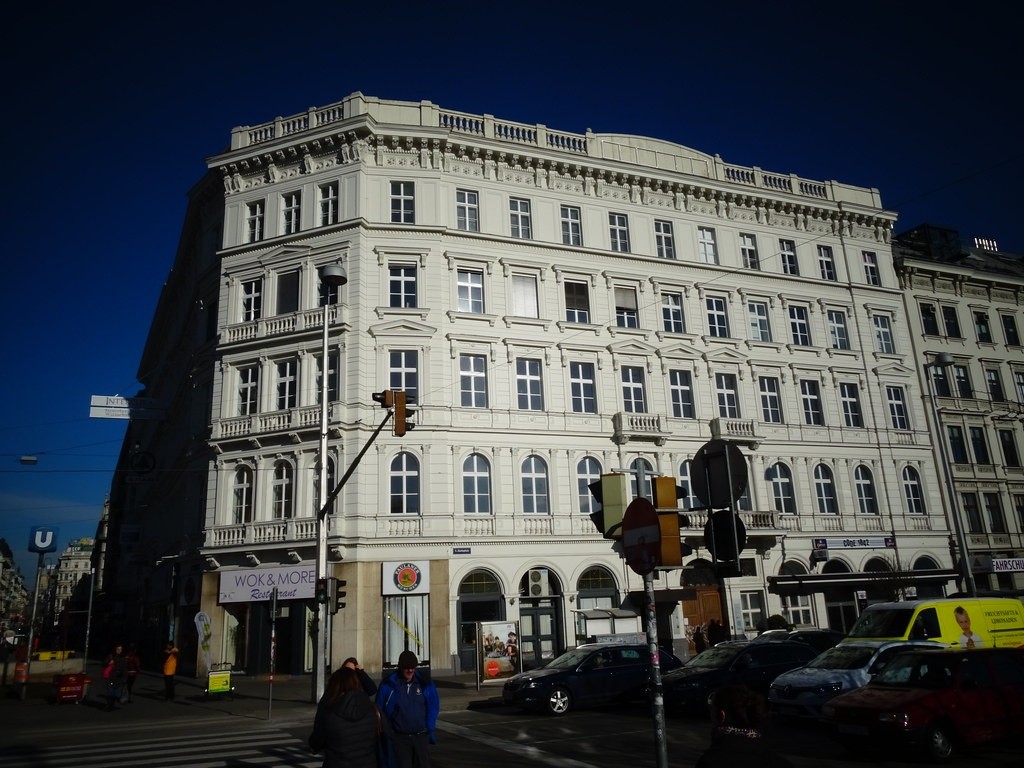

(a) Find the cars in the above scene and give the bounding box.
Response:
[765,638,952,731]
[642,637,819,724]
[752,628,848,655]
[820,647,1024,764]
[502,643,686,716]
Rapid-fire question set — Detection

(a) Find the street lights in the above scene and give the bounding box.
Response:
[922,352,977,598]
[309,264,348,706]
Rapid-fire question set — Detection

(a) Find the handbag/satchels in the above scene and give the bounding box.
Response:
[102,655,114,679]
[372,704,382,735]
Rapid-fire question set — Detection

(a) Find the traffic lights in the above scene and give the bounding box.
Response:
[649,476,693,571]
[315,579,328,605]
[330,577,347,616]
[394,391,415,437]
[587,472,628,538]
[372,390,394,408]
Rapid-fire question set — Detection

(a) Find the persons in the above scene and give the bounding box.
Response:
[120,644,139,703]
[376,650,439,768]
[102,646,124,711]
[309,667,376,768]
[486,632,517,657]
[343,658,377,696]
[163,640,179,702]
[2,637,28,661]
[954,607,982,648]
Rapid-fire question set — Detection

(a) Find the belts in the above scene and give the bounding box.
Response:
[394,731,428,737]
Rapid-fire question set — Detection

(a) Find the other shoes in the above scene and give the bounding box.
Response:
[127,700,133,703]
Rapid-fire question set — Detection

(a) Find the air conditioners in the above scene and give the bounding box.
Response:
[528,569,548,596]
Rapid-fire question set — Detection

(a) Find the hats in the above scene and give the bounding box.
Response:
[397,651,418,667]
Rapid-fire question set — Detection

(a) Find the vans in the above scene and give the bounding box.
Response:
[840,597,1024,650]
